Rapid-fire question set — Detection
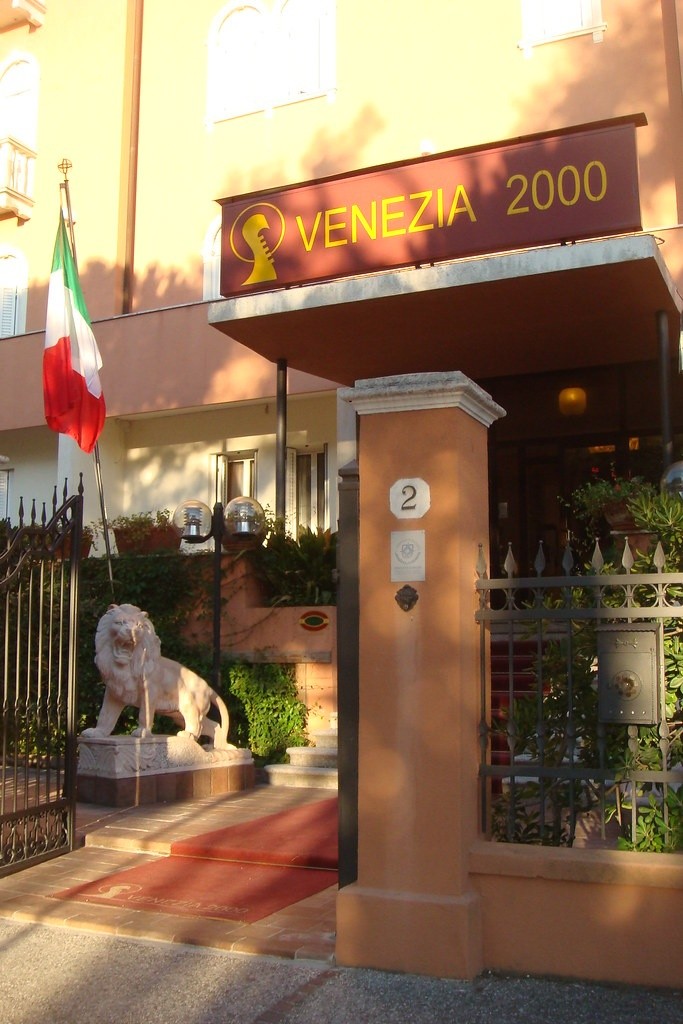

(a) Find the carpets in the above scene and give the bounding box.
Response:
[44,639,566,924]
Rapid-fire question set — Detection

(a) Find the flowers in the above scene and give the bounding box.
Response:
[555,460,659,533]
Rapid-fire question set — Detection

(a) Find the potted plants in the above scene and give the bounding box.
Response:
[98,507,184,556]
[19,522,98,559]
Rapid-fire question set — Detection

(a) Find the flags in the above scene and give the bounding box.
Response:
[42,209,105,452]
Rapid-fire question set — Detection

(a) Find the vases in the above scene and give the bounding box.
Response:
[602,496,644,531]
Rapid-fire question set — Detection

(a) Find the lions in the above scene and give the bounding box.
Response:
[80,603,238,751]
